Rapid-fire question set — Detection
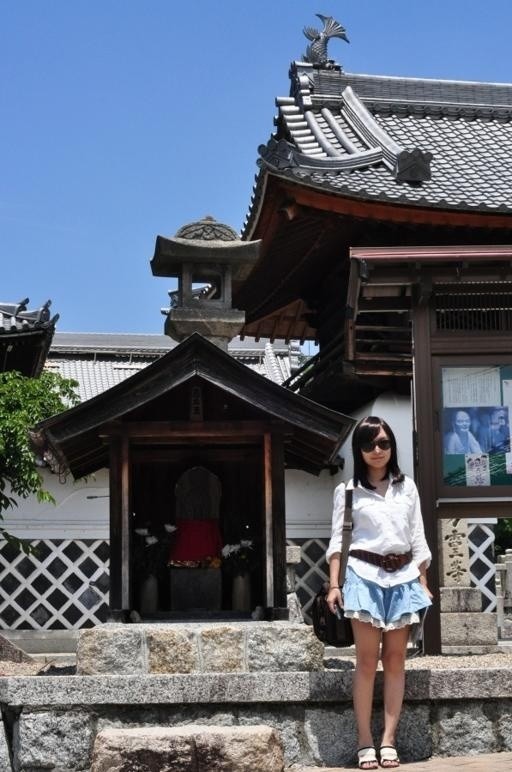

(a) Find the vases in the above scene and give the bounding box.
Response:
[232,574,251,614]
[141,571,159,612]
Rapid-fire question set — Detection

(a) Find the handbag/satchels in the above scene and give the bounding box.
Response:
[308,589,359,653]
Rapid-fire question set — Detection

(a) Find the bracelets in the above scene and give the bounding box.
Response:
[329,587,341,590]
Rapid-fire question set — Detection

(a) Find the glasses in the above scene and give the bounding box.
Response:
[358,439,394,454]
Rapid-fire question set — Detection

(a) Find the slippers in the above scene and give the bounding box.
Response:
[378,743,401,768]
[355,744,379,770]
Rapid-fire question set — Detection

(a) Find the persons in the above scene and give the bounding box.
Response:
[477,409,507,451]
[325,414,436,771]
[475,457,481,470]
[467,457,475,469]
[481,455,488,471]
[442,410,483,454]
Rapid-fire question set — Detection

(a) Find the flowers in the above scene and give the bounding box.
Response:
[134,523,177,573]
[220,540,255,573]
